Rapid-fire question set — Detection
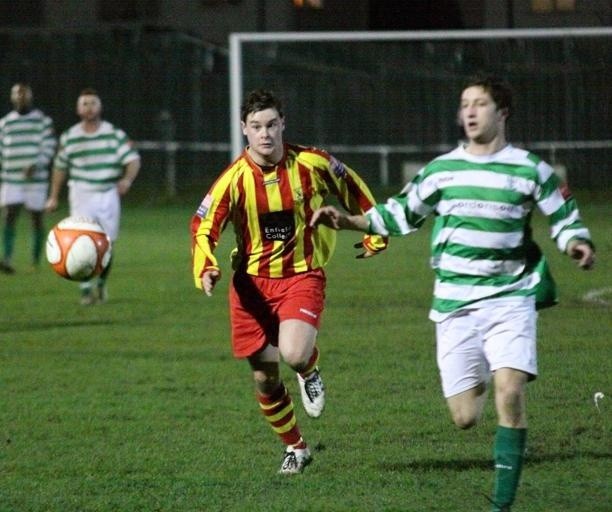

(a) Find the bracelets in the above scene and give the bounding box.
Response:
[122,178,132,187]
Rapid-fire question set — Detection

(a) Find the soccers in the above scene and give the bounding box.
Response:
[45,216,111,281]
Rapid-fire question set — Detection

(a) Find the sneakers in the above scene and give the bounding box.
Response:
[82,284,108,306]
[280,445,311,474]
[296,372,326,419]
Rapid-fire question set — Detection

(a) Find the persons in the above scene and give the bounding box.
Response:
[308,72,599,512]
[190,90,391,477]
[44,88,142,307]
[1,80,58,273]
